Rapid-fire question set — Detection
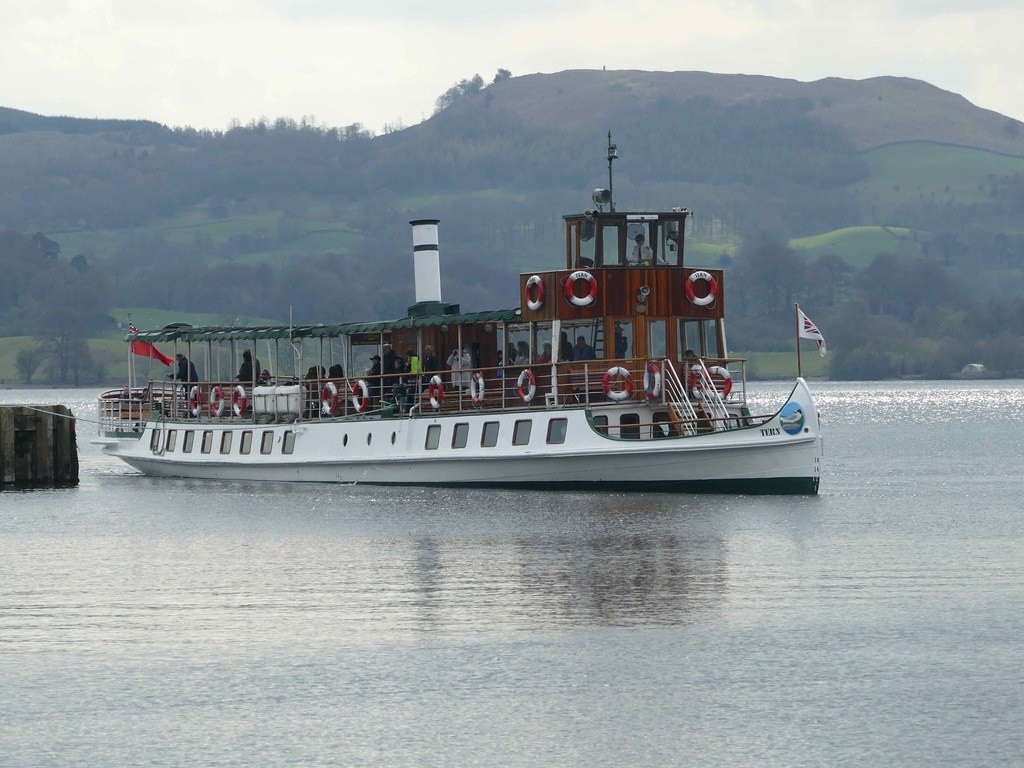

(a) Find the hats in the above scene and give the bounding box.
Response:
[370,356,381,361]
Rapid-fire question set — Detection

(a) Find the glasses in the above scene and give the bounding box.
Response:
[262,374,267,376]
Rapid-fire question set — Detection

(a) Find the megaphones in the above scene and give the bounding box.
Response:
[640,286,650,296]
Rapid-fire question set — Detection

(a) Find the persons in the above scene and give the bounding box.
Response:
[235,348,271,388]
[382,343,436,413]
[363,355,381,414]
[284,364,343,418]
[629,234,665,264]
[447,346,472,410]
[685,350,699,357]
[571,336,596,359]
[615,326,628,359]
[561,332,573,361]
[497,341,552,378]
[164,353,198,418]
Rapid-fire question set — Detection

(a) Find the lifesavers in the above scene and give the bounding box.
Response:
[352,380,369,412]
[470,373,484,404]
[210,386,225,417]
[565,270,598,306]
[322,382,338,414]
[691,364,706,399]
[602,366,634,401]
[524,275,544,311]
[643,364,661,398]
[190,386,202,416]
[429,375,443,408]
[684,270,717,306]
[704,366,733,400]
[517,369,537,403]
[232,384,247,417]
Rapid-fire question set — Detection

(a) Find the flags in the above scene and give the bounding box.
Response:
[129,321,176,366]
[797,307,826,357]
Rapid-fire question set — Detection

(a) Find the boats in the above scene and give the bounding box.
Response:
[951,363,988,380]
[89,123,824,497]
